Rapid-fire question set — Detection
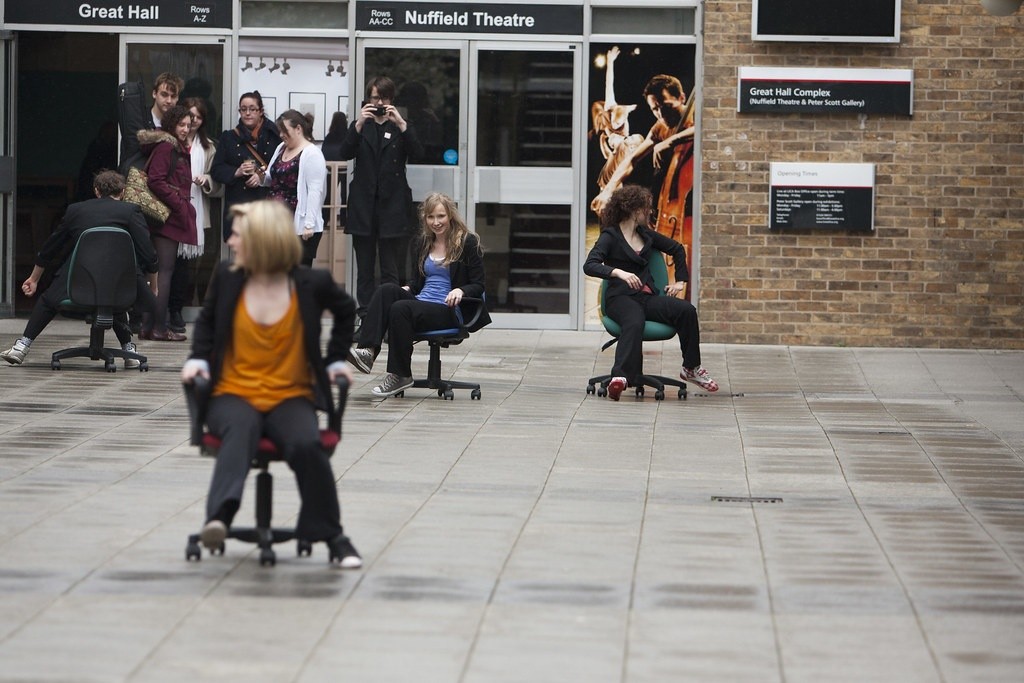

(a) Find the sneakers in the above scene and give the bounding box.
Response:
[372,372,414,398]
[680,365,719,392]
[607,377,627,401]
[348,348,375,375]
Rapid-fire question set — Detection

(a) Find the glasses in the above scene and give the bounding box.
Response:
[238,107,261,114]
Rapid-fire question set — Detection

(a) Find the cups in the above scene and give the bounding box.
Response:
[243,160,255,175]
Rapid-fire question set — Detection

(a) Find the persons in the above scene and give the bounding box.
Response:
[583,185,720,401]
[590,46,697,222]
[119,71,348,340]
[180,198,362,568]
[339,75,425,343]
[0,170,158,369]
[347,192,492,397]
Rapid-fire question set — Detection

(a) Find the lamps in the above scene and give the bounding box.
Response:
[326,60,334,76]
[255,57,266,71]
[268,58,280,73]
[241,57,252,72]
[281,58,290,75]
[337,61,346,77]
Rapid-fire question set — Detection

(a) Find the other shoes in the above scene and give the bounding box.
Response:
[123,348,140,368]
[201,516,228,548]
[329,537,362,568]
[352,326,363,343]
[0,338,31,365]
[137,327,151,339]
[383,330,389,343]
[168,326,186,333]
[150,328,187,341]
[170,309,186,327]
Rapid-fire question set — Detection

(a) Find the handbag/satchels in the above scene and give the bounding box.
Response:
[124,168,180,223]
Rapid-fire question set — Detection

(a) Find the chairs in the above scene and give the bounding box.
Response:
[394,292,485,401]
[183,375,348,567]
[587,250,688,401]
[51,227,148,374]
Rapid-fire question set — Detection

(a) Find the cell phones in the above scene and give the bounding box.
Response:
[370,108,386,116]
